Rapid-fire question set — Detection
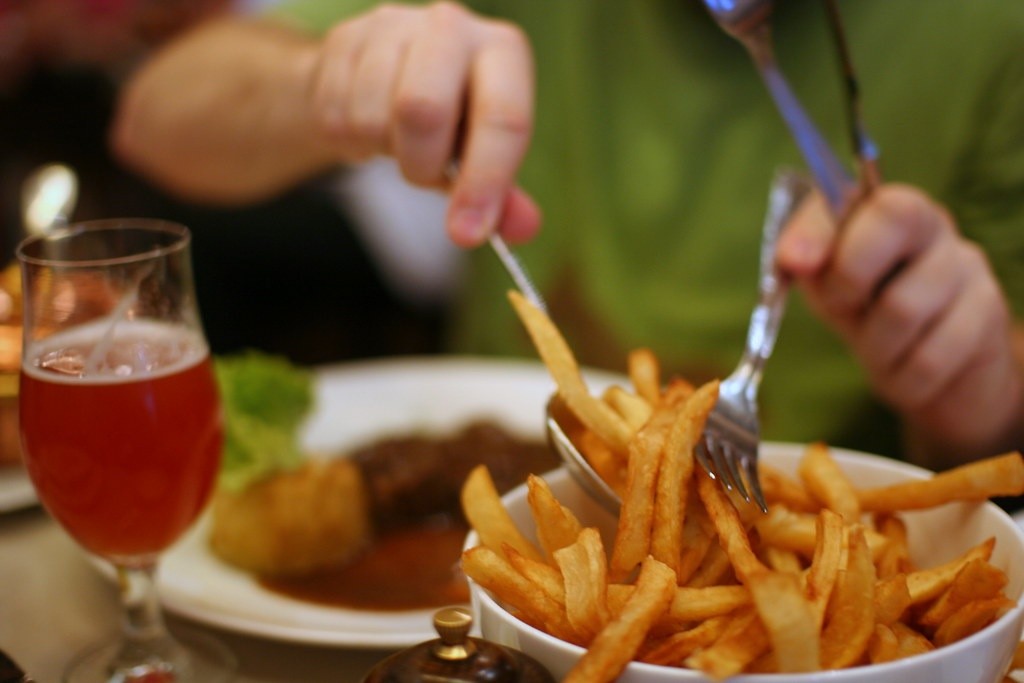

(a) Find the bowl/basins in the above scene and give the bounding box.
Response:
[460,440,1024,683]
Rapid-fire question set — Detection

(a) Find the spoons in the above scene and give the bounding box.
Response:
[444,163,623,519]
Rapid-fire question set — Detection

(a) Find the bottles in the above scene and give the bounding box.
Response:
[361,606,556,683]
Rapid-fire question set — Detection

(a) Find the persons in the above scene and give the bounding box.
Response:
[113,0,1024,515]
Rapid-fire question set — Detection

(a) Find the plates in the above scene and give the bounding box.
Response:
[84,354,664,647]
[0,459,40,513]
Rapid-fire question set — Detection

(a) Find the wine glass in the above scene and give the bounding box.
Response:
[18,217,238,683]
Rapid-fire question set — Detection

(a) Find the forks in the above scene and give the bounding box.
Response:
[691,161,794,514]
